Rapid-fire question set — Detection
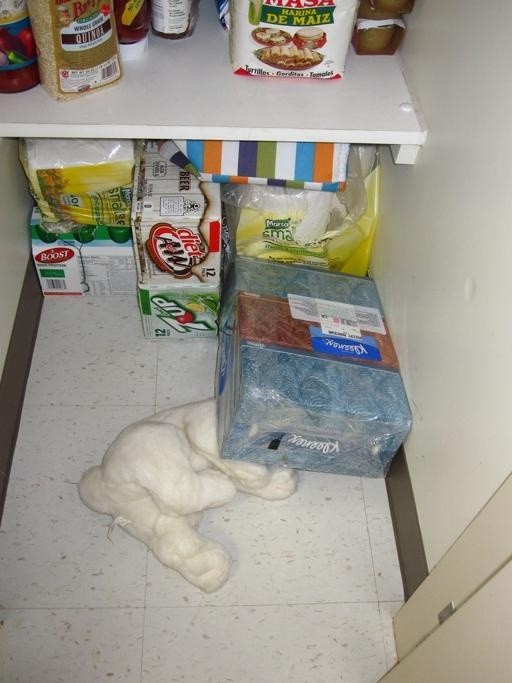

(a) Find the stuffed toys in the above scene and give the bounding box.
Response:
[79,397,301,594]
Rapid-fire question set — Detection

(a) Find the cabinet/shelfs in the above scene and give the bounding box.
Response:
[0,0,424,270]
